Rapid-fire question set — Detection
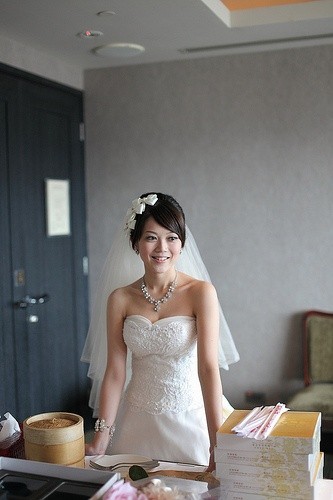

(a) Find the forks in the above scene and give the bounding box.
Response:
[89,459,161,471]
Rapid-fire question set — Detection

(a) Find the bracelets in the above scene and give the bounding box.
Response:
[93,418,118,435]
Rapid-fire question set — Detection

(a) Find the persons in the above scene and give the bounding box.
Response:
[80,192,241,472]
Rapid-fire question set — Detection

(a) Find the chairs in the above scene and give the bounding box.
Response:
[285,309,333,432]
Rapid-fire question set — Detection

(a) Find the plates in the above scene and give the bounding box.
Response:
[128,471,220,490]
[92,455,157,471]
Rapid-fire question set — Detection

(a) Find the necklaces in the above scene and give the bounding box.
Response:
[140,272,178,311]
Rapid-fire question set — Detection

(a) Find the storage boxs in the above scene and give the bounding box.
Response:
[214,410,324,500]
[0,457,121,500]
